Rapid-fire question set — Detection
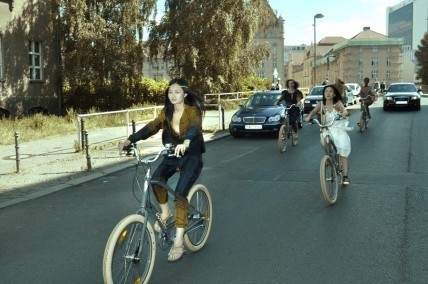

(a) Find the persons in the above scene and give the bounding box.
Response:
[325,77,331,85]
[380,81,385,92]
[356,77,375,127]
[119,77,206,263]
[334,78,351,96]
[271,78,281,90]
[304,85,351,184]
[276,78,303,139]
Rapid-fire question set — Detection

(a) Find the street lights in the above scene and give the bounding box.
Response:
[313,13,324,86]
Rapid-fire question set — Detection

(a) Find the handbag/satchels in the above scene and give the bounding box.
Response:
[318,100,326,129]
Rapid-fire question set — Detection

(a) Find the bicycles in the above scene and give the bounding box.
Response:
[302,113,351,206]
[356,94,372,132]
[102,141,213,284]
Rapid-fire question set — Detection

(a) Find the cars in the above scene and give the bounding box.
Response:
[304,85,326,114]
[382,82,423,112]
[346,82,362,105]
[229,89,291,139]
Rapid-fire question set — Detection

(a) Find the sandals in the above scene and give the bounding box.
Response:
[153,212,173,232]
[167,241,185,261]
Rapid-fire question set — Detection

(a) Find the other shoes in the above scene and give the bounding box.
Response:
[342,177,350,185]
[294,132,298,139]
